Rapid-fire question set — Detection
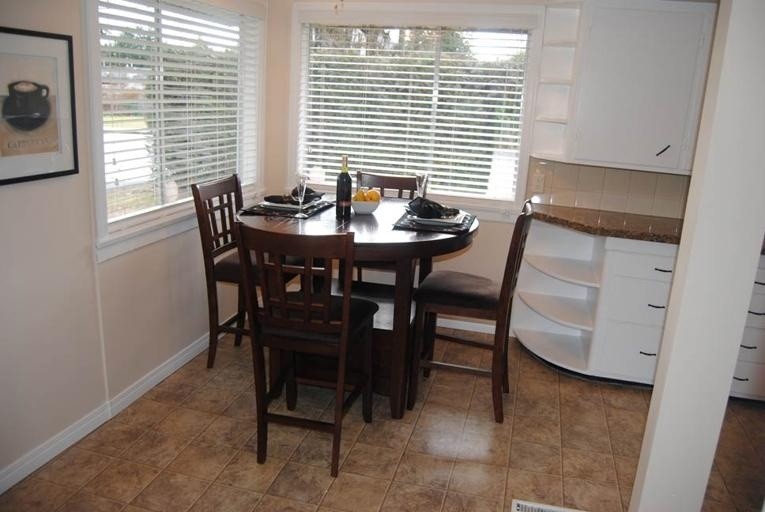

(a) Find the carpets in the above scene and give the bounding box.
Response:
[509,496,589,511]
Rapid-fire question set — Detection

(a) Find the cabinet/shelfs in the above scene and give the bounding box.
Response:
[566,0,721,176]
[516,2,582,164]
[509,154,607,379]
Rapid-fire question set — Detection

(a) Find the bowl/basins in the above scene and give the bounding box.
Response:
[350,199,380,216]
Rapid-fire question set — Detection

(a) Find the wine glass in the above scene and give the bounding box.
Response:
[293,172,310,220]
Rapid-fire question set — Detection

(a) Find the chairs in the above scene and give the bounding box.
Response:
[405,194,534,422]
[224,221,380,478]
[191,173,302,368]
[333,169,433,307]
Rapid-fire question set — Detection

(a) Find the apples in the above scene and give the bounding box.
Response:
[354,189,379,201]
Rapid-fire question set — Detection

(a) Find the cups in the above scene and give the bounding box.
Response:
[7,81,50,112]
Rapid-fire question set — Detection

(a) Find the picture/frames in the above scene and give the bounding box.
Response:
[0,25,79,186]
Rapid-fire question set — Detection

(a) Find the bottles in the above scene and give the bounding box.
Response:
[334,153,353,222]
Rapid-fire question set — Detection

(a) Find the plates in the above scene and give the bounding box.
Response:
[2,98,50,131]
[406,209,468,227]
[259,195,325,212]
